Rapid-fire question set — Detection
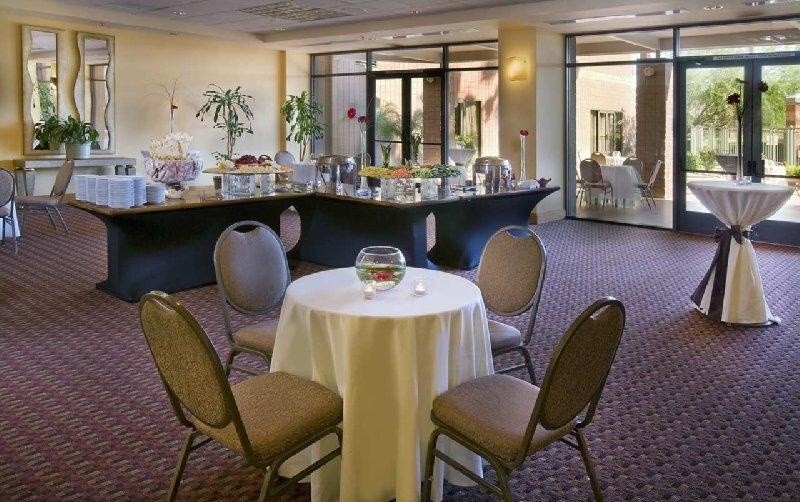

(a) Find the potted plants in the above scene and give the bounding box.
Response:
[50,116,99,159]
[34,114,67,149]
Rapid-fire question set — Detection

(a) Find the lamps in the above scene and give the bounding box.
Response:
[506,56,528,81]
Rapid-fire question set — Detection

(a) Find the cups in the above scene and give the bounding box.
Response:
[306,180,314,190]
[414,279,427,294]
[222,173,270,197]
[318,178,323,189]
[362,281,376,299]
[730,176,751,186]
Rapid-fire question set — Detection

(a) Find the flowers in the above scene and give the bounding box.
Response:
[519,129,529,181]
[347,108,369,175]
[724,78,768,177]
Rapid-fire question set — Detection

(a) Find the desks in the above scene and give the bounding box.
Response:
[13,158,136,197]
[448,149,476,186]
[63,180,560,303]
[688,181,794,327]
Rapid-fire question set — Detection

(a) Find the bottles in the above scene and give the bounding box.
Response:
[380,178,416,204]
[421,178,439,199]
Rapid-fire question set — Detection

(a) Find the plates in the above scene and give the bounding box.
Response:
[75,175,165,209]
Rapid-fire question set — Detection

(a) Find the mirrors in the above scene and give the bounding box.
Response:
[73,31,116,154]
[21,24,67,156]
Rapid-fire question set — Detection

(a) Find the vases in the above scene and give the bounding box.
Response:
[520,135,526,184]
[360,123,368,192]
[736,119,744,185]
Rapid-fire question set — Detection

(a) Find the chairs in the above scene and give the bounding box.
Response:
[1,158,75,255]
[576,152,663,210]
[477,225,547,387]
[213,221,291,376]
[139,290,343,501]
[422,296,625,502]
